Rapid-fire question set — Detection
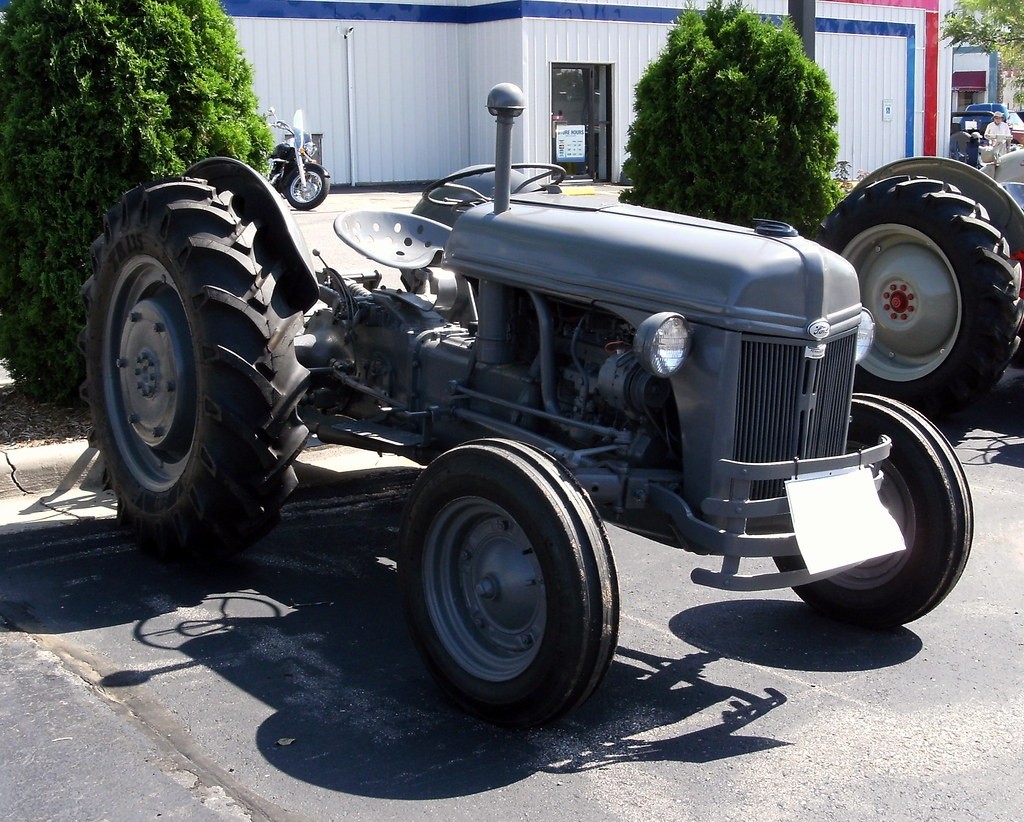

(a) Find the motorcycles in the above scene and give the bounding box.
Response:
[262,105,330,211]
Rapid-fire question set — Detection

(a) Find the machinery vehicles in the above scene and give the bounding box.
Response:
[68,79,974,729]
[809,147,1024,423]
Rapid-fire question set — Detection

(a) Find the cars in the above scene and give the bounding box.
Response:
[958,102,1024,146]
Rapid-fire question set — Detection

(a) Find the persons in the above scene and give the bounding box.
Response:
[984,111,1012,146]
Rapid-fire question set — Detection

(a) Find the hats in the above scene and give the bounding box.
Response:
[971,131,983,140]
[991,111,1005,120]
